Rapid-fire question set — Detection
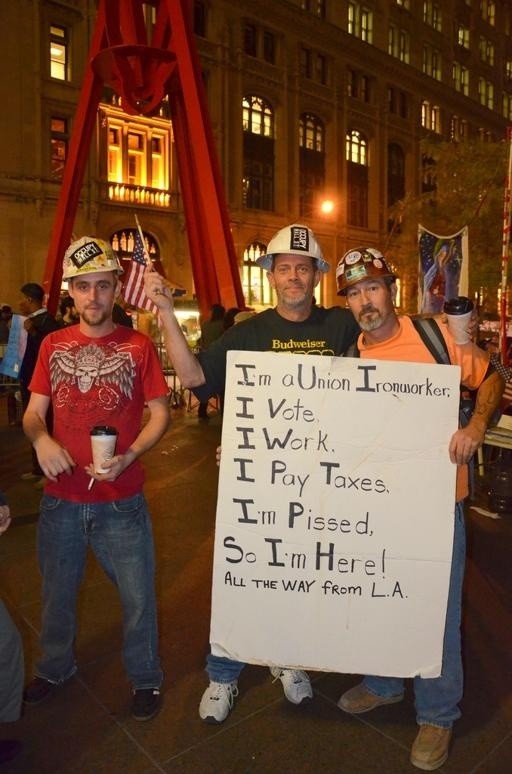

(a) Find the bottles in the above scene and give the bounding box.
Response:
[87,427,119,474]
[444,296,473,344]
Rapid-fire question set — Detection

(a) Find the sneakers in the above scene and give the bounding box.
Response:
[197,676,239,726]
[19,471,43,482]
[32,476,48,490]
[278,669,314,706]
[408,723,453,772]
[128,667,165,723]
[0,504,12,534]
[336,679,405,715]
[17,655,79,710]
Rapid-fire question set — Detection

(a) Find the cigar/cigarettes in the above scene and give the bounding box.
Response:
[91,477,93,491]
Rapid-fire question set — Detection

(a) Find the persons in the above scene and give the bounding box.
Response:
[337,246,504,771]
[57,296,80,331]
[144,224,479,726]
[0,503,20,763]
[0,305,13,343]
[199,302,224,420]
[20,282,56,487]
[23,236,173,724]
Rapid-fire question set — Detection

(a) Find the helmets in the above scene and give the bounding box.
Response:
[62,235,126,282]
[254,223,330,274]
[334,246,399,298]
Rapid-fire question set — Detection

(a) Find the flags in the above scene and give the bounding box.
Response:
[123,233,160,329]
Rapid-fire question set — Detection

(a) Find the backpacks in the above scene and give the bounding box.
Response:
[456,378,502,430]
